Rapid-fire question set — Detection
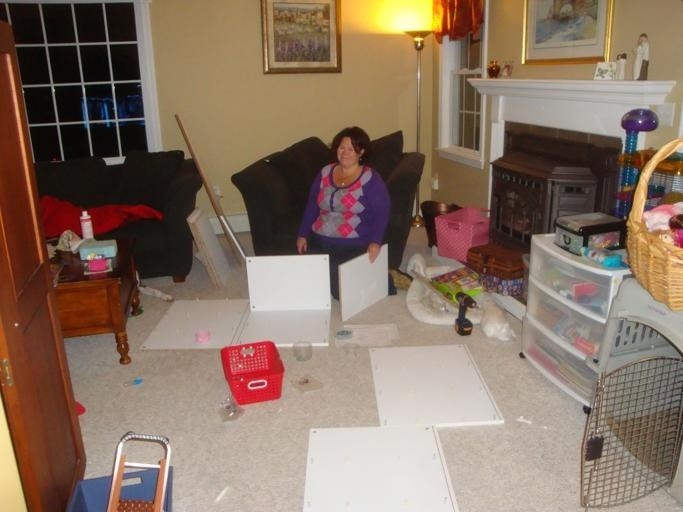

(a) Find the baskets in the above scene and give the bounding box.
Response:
[624,137,683,311]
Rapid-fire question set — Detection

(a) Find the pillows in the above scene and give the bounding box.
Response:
[40,149,185,211]
[364,130,407,177]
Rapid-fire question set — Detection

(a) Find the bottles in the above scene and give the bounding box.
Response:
[79,210,93,240]
[578,246,610,264]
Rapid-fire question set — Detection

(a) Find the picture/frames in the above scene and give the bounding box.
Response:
[260,0,342,75]
[518,1,614,66]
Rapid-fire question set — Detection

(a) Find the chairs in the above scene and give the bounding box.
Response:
[231,141,424,273]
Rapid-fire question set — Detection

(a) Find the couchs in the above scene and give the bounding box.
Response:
[34,157,204,285]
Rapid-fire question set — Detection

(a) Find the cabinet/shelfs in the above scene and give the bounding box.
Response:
[517,231,631,417]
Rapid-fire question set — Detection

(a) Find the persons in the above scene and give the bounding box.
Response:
[614,53,627,81]
[632,32,650,80]
[296,126,397,300]
